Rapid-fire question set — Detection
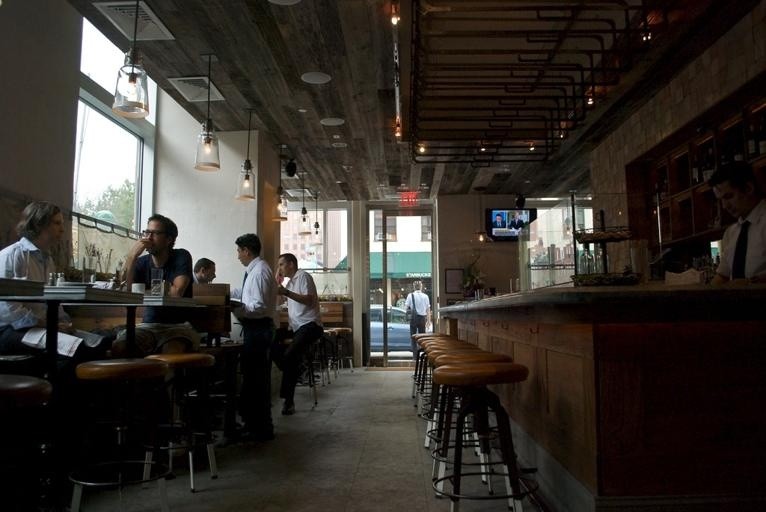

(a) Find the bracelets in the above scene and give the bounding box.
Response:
[744,277,751,286]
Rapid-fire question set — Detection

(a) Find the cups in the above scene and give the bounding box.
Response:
[132,283,145,294]
[475,291,478,300]
[510,278,520,293]
[477,289,484,300]
[83,256,98,283]
[151,268,163,296]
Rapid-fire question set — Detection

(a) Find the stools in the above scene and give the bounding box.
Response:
[68,358,170,512]
[283,326,354,409]
[146,354,218,493]
[411,332,540,512]
[0,373,52,512]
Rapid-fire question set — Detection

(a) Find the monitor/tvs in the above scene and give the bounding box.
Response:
[485,208,537,242]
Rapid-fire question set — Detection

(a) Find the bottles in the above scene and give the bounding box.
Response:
[116,258,128,285]
[48,272,56,286]
[57,273,65,287]
[594,244,610,273]
[580,243,594,274]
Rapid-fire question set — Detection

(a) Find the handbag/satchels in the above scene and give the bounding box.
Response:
[406,309,417,321]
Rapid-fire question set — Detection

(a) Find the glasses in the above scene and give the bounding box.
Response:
[49,221,64,225]
[143,231,170,237]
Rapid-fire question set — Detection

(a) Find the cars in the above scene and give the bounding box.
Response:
[369,305,431,350]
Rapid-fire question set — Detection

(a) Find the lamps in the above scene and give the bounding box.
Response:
[236,111,256,200]
[194,56,220,172]
[271,148,288,221]
[312,193,322,247]
[298,169,312,235]
[112,0,149,120]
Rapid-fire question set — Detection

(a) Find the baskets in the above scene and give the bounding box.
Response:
[572,226,633,243]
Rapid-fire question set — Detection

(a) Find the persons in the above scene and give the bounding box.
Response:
[0,201,107,392]
[403,280,431,361]
[192,257,216,283]
[273,253,324,416]
[111,213,201,457]
[705,159,765,287]
[509,211,524,230]
[229,232,280,443]
[492,214,506,228]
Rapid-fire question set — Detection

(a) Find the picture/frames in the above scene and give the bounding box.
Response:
[445,268,465,294]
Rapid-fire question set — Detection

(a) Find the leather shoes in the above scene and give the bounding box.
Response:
[282,402,295,414]
[237,427,273,441]
[294,364,307,385]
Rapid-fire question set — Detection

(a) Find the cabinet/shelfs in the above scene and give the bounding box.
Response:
[645,97,765,282]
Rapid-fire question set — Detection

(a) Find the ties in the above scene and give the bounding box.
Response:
[241,271,248,303]
[499,224,500,228]
[733,221,750,280]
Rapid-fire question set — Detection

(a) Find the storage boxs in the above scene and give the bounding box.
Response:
[1,278,45,296]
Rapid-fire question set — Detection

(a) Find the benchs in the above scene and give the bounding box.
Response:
[0,354,34,373]
[279,302,344,323]
[197,283,246,349]
[64,284,225,357]
